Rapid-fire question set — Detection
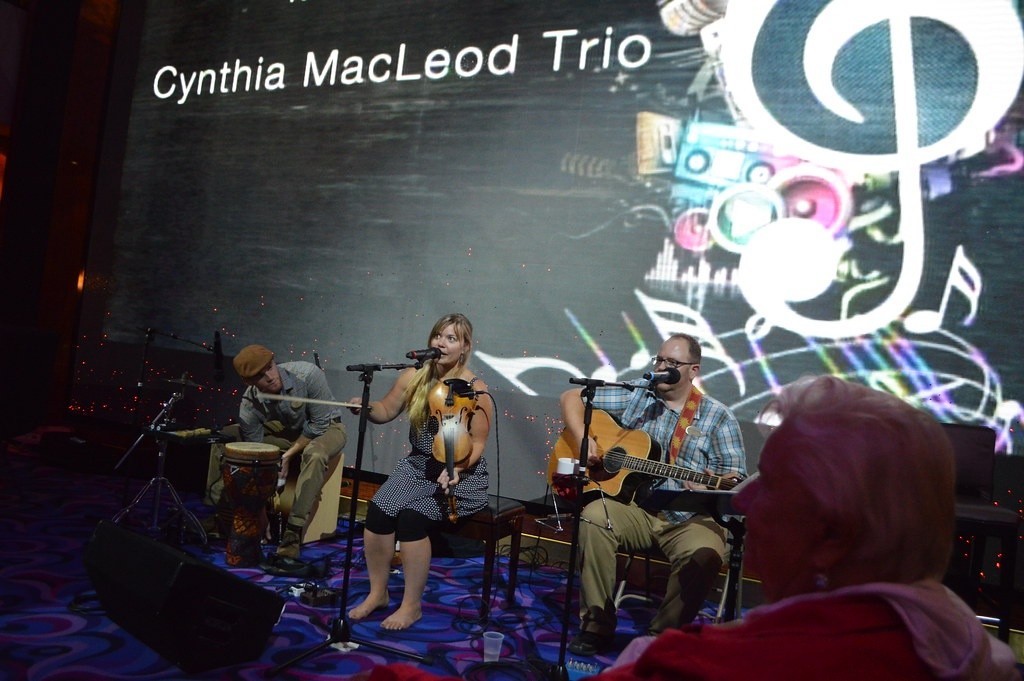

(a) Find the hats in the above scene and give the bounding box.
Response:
[233,344,274,378]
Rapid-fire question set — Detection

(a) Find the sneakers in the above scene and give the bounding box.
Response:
[567,628,619,657]
[276,529,301,559]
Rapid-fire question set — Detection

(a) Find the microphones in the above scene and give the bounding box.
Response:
[213,330,225,382]
[405,347,442,360]
[643,367,681,384]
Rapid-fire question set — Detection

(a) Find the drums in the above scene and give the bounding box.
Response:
[223,442,281,566]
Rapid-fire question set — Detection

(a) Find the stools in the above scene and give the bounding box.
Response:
[613,547,731,627]
[424,495,525,621]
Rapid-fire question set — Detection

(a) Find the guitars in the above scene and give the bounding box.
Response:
[548,408,742,506]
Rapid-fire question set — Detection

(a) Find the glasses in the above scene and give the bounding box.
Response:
[651,357,698,369]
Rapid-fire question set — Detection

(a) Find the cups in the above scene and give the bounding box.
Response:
[483,631,504,662]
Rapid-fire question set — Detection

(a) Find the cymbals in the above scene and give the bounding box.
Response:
[159,377,208,391]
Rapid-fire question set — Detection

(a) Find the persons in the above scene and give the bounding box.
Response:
[562,330,749,657]
[349,313,494,631]
[579,376,1024,681]
[234,342,349,560]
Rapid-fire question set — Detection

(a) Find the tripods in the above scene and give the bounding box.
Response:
[110,382,213,555]
[272,360,434,672]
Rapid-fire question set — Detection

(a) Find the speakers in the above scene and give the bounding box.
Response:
[82,520,286,672]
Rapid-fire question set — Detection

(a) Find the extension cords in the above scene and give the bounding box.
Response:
[289,582,319,597]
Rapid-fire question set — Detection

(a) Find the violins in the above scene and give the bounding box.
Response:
[428,378,479,524]
